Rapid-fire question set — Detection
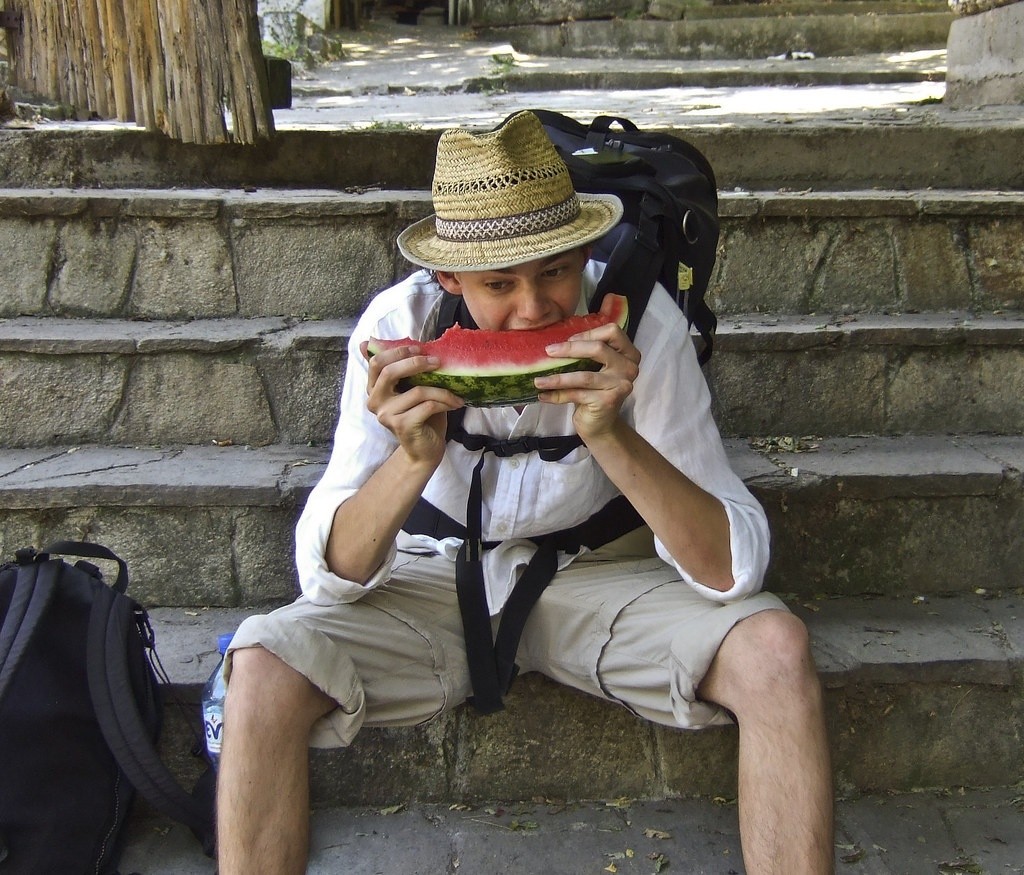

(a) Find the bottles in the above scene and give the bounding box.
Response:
[201,633,236,776]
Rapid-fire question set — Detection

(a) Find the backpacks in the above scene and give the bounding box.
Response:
[429,106,719,710]
[0,540,192,875]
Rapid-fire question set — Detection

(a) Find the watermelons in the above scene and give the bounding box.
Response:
[366,293,629,408]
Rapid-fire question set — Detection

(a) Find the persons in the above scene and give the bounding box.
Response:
[217,111,836,875]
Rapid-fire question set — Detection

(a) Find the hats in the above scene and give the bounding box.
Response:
[394,110,625,273]
